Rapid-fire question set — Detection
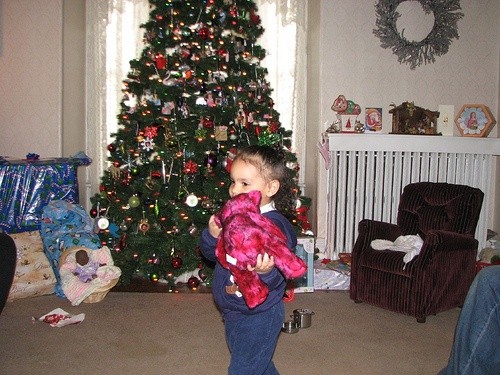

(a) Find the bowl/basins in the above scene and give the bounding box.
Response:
[281,322,301,333]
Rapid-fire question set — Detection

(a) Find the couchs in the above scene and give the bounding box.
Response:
[351,182,484,323]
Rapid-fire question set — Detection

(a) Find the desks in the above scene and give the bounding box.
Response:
[0,157,92,236]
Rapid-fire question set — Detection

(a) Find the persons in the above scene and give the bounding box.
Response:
[467,112,479,130]
[73,250,107,283]
[444,264,500,375]
[200,145,298,375]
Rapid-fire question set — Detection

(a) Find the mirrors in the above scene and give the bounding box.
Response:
[454,103,496,138]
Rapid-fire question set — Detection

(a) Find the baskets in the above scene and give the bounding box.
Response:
[58,245,121,302]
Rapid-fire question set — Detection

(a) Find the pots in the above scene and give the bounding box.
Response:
[290,309,315,328]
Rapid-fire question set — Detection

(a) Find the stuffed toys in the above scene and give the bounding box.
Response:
[214,191,308,311]
